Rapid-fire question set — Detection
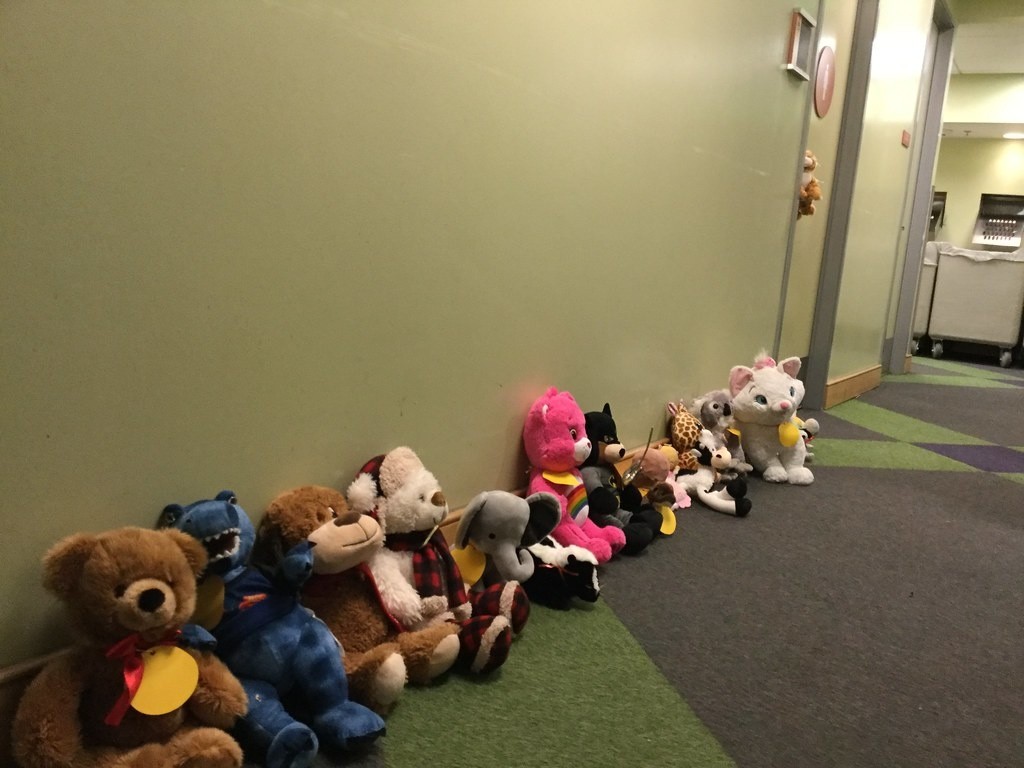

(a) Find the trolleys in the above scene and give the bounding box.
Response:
[927,242,1024,367]
[906,257,938,354]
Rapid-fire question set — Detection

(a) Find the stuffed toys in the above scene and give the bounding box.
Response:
[796,150,822,219]
[447,490,561,592]
[627,448,678,535]
[729,352,816,485]
[657,442,692,511]
[794,415,820,461]
[672,429,752,517]
[581,403,663,556]
[691,387,753,475]
[527,536,600,607]
[522,384,628,565]
[345,446,531,675]
[160,490,386,768]
[259,486,463,713]
[667,398,738,483]
[10,525,248,768]
